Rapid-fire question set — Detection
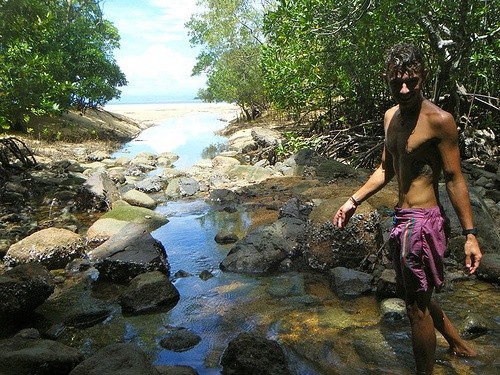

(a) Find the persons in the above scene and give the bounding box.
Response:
[333,42,484,375]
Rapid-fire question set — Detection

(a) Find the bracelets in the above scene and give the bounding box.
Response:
[460,227,479,239]
[349,196,362,208]
